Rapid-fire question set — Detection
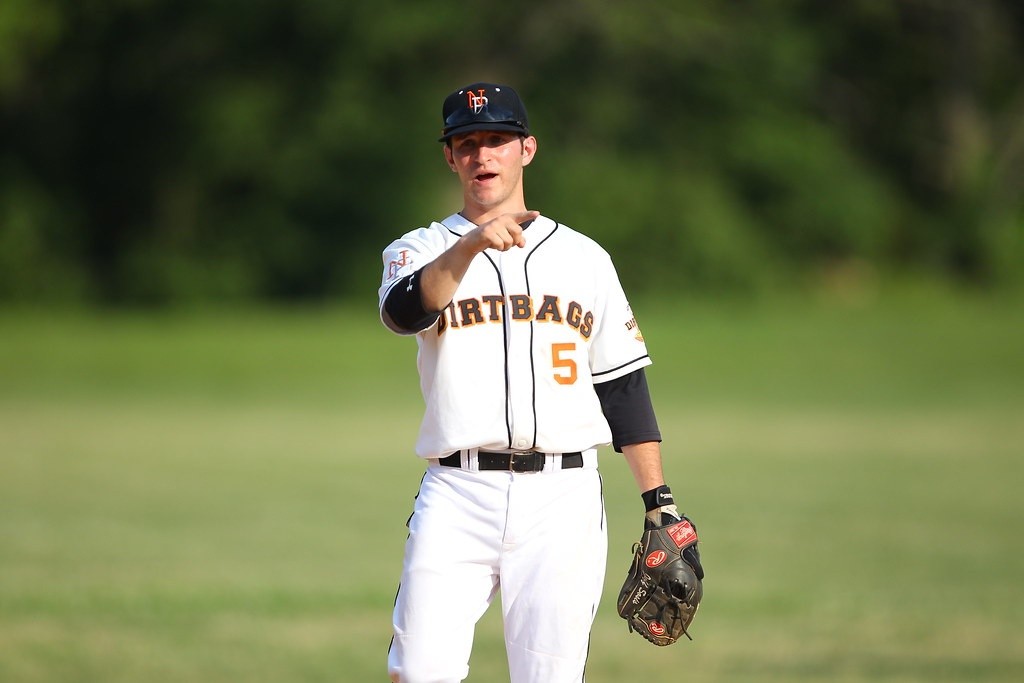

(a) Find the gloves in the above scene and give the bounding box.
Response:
[641,485,705,579]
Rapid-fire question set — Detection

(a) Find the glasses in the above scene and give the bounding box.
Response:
[441,108,529,133]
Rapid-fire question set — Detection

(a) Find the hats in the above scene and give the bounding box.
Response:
[438,82,529,142]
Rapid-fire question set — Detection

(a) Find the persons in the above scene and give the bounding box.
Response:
[378,83,703,682]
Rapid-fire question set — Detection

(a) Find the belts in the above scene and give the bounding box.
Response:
[439,450,583,474]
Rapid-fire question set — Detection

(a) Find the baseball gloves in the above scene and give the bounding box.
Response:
[612,512,706,649]
